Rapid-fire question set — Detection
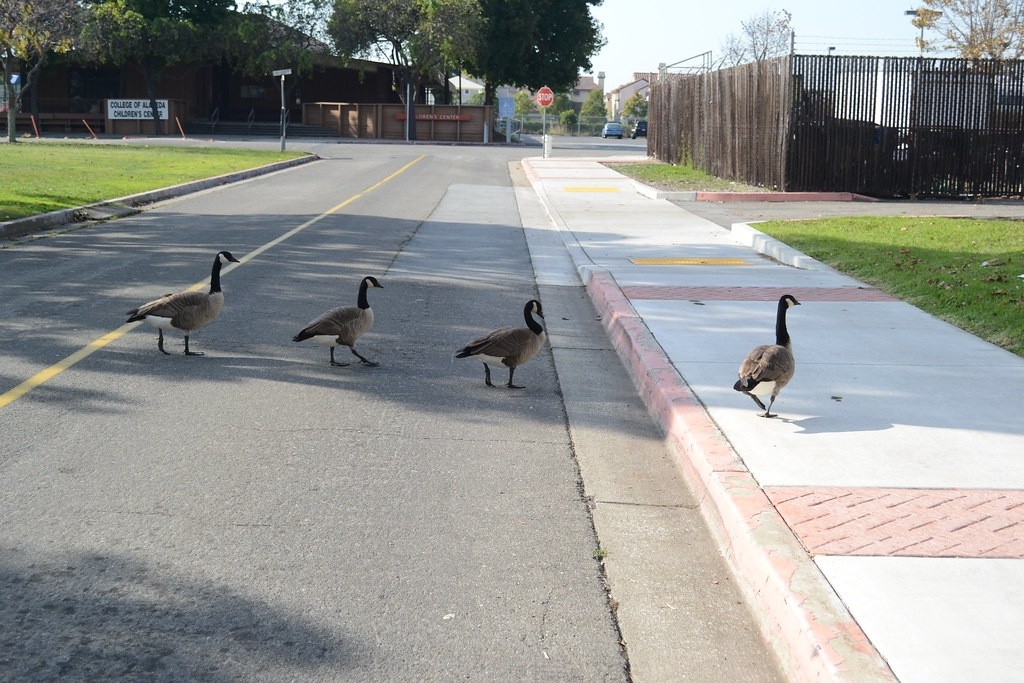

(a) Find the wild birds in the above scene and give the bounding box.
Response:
[733,295,802,418]
[454,299,547,388]
[291,276,384,366]
[125,251,240,355]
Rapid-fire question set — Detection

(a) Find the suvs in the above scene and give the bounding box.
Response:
[602,123,622,139]
[631,121,647,140]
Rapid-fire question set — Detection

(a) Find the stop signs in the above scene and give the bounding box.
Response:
[537,87,553,107]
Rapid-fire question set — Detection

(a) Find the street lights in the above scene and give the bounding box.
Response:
[273,69,291,152]
